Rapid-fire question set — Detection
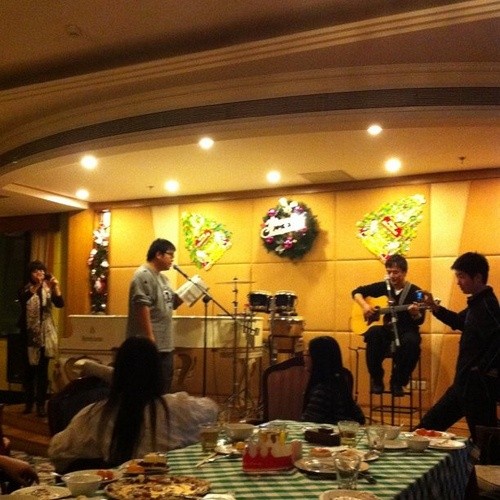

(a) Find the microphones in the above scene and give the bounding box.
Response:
[384,276,391,289]
[172,265,187,278]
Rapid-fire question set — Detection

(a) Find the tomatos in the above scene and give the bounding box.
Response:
[97,470,113,480]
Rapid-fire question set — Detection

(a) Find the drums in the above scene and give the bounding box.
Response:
[248,290,273,313]
[270,290,297,316]
[267,317,305,356]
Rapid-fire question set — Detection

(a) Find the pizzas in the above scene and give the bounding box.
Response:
[106,476,209,500]
[310,449,332,458]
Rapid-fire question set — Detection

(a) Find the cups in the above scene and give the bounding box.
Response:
[330,449,361,491]
[365,425,384,453]
[197,422,220,452]
[337,420,358,448]
[407,436,429,452]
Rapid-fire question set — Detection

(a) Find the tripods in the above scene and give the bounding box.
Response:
[223,286,260,413]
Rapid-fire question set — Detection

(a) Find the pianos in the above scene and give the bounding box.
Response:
[47,315,263,409]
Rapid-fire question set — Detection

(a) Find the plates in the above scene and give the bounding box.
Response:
[373,436,409,450]
[425,437,467,449]
[11,486,72,500]
[119,458,171,475]
[61,469,124,485]
[413,429,457,439]
[294,449,369,476]
[319,489,383,500]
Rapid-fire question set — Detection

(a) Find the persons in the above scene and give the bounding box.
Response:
[302,336,355,424]
[18,260,64,417]
[47,336,221,470]
[352,254,426,397]
[409,252,500,435]
[126,239,183,393]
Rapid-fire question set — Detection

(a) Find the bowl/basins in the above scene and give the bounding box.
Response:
[225,423,254,440]
[61,473,102,496]
[379,427,401,440]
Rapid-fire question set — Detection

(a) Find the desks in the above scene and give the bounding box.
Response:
[92,420,476,500]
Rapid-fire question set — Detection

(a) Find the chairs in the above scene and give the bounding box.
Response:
[47,375,111,482]
[263,359,353,422]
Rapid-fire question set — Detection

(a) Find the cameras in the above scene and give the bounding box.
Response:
[303,426,340,445]
[43,273,52,280]
[416,290,425,300]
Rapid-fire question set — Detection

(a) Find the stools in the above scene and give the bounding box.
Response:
[369,351,422,428]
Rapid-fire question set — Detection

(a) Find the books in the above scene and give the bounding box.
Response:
[175,275,208,307]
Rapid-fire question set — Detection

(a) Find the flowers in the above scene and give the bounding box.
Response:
[86,221,111,312]
[259,198,319,260]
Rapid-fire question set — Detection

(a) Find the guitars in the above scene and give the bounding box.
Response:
[351,295,440,335]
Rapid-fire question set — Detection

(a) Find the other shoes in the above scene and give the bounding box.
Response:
[36,406,47,417]
[370,376,385,394]
[20,403,35,414]
[389,380,404,396]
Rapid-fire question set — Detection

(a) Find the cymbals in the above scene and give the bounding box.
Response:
[215,278,256,285]
[215,312,256,317]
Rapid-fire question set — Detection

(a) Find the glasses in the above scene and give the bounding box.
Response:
[165,252,174,257]
